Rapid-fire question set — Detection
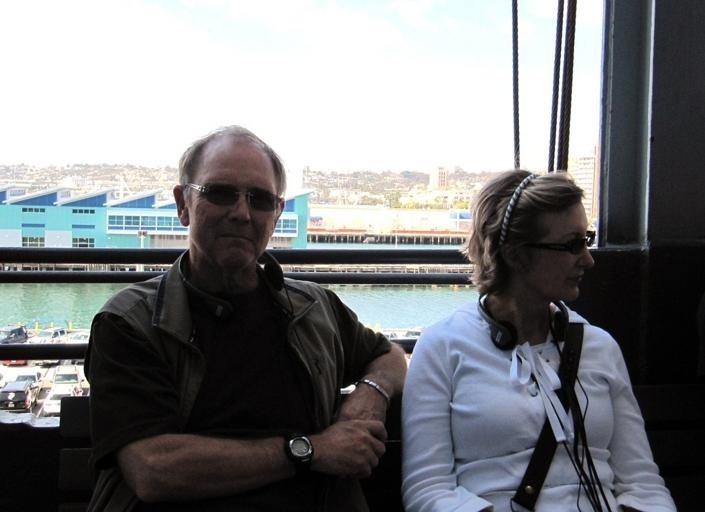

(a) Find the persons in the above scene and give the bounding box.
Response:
[83,124,409,511]
[400,167,679,511]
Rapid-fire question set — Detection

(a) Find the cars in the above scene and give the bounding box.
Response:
[1,322,93,416]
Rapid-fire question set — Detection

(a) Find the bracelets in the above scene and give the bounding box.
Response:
[355,379,392,408]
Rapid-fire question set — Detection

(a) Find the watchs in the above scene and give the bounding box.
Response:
[283,431,314,476]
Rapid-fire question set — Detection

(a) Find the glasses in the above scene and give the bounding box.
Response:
[191,183,283,211]
[532,231,596,254]
